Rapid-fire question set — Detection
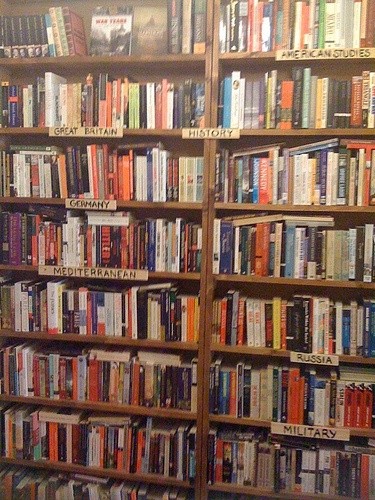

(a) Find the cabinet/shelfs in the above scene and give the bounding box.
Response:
[212,0,374,498]
[0,1,214,500]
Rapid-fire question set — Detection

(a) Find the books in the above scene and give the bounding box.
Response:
[0,0,375,500]
